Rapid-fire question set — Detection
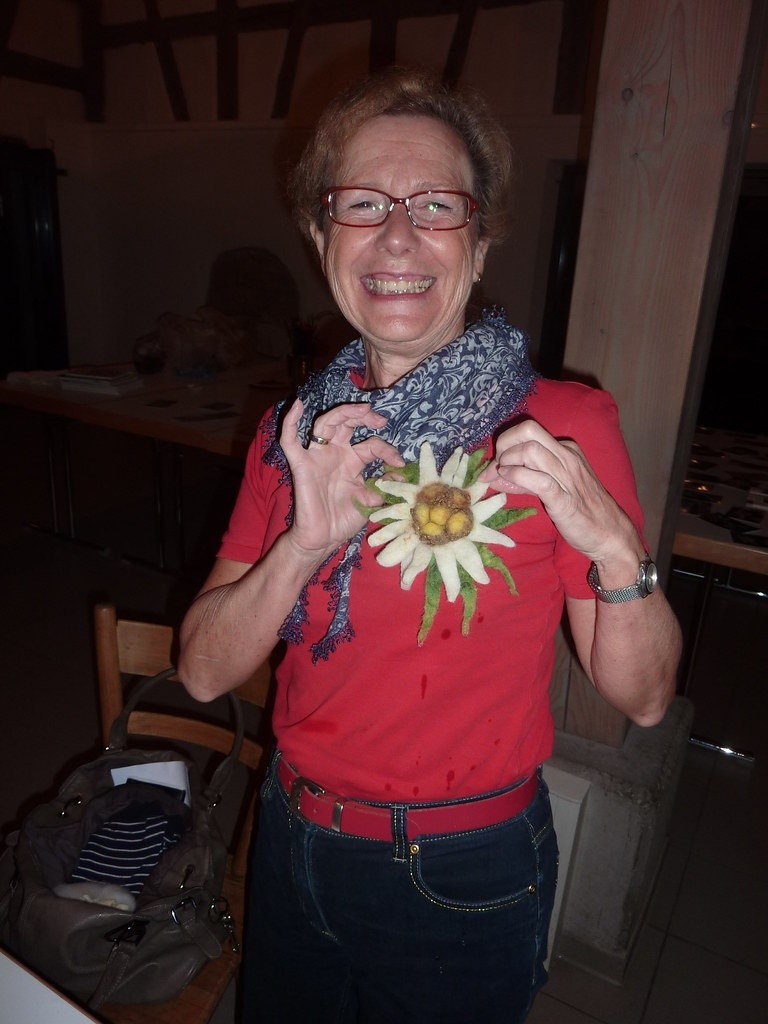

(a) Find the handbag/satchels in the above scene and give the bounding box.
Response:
[2,666,246,1009]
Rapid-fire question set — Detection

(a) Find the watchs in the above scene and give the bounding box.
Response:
[588,550,658,604]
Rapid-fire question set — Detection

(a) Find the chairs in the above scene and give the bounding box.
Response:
[57,599,276,1024]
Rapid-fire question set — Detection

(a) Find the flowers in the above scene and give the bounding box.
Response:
[354,440,540,651]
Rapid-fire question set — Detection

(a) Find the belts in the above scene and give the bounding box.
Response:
[274,757,544,840]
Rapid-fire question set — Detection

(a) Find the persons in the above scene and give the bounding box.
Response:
[177,67,684,1024]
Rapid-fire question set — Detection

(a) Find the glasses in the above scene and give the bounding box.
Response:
[321,185,480,231]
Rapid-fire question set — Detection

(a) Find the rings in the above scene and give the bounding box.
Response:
[311,435,328,445]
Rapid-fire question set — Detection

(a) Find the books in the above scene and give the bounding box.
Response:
[55,366,145,395]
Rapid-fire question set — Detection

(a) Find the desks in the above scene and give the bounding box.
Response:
[0,360,298,585]
[660,422,766,763]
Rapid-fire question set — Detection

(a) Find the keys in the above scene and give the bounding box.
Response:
[220,912,241,955]
